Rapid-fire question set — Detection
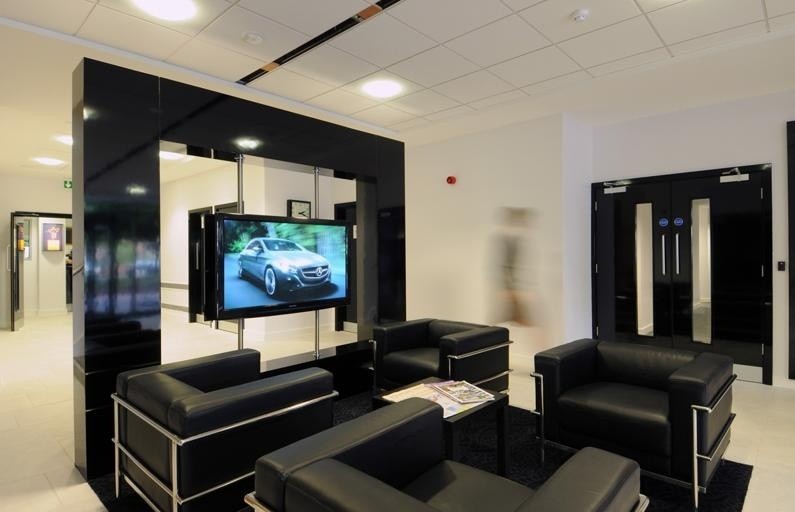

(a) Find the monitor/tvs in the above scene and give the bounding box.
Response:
[200,212,215,322]
[213,213,353,320]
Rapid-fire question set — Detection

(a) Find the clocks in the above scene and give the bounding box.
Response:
[286,199,312,218]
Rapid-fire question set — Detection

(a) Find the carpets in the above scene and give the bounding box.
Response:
[86,371,756,512]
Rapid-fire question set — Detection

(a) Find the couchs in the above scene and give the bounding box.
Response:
[365,316,513,407]
[529,337,735,497]
[113,345,342,509]
[241,394,651,512]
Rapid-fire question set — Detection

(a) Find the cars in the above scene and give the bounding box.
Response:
[236,237,332,298]
